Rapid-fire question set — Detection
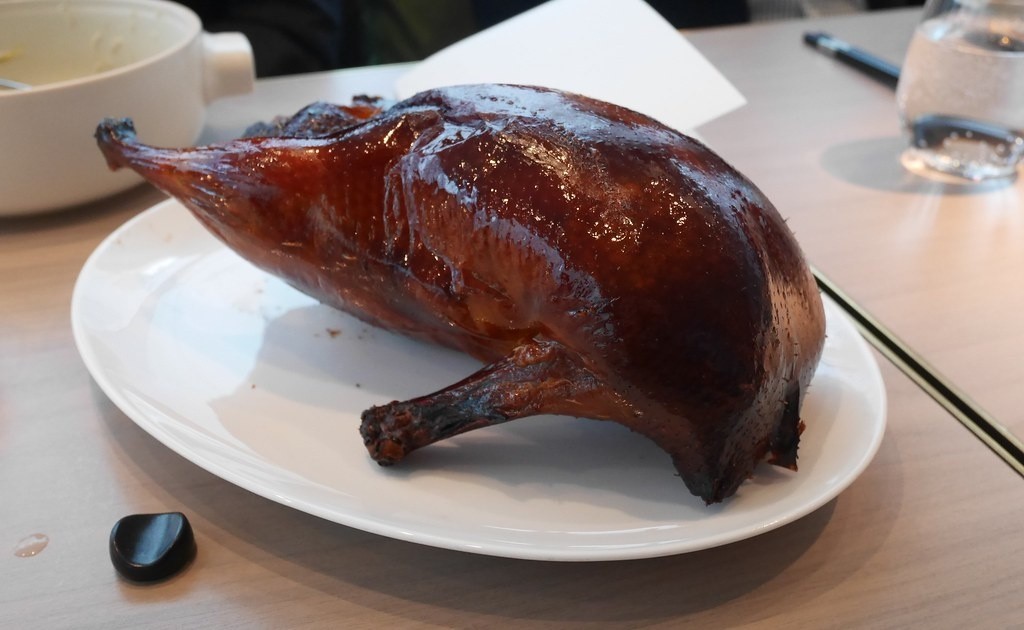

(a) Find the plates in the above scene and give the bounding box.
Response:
[71,193,888,561]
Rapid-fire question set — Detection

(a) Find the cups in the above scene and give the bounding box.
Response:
[895,0,1024,187]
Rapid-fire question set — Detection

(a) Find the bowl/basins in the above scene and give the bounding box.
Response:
[0,0,255,216]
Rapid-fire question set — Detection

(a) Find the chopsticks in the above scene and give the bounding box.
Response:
[811,264,1024,480]
[802,32,902,92]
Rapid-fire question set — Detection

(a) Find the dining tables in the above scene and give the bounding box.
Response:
[1,0,1024,630]
